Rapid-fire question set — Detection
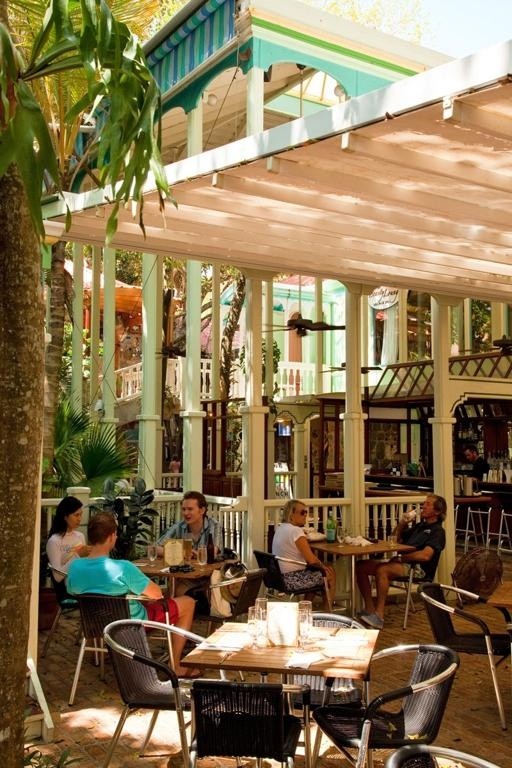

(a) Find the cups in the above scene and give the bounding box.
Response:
[404,508,424,522]
[388,536,397,547]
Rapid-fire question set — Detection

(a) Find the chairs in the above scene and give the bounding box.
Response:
[101,619,205,768]
[311,643,460,768]
[260,612,370,757]
[417,581,512,731]
[68,595,175,705]
[369,542,445,630]
[386,744,500,768]
[40,564,100,666]
[187,680,324,767]
[198,567,267,637]
[253,549,333,613]
[318,478,510,555]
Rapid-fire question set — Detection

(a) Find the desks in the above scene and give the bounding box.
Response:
[310,540,416,620]
[179,622,381,768]
[131,558,239,599]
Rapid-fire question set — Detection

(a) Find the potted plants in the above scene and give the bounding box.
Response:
[406,463,420,476]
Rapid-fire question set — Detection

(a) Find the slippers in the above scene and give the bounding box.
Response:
[178,668,204,679]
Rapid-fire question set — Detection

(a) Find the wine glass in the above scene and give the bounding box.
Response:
[336,527,344,547]
[146,543,157,567]
[183,533,193,572]
[247,606,262,649]
[296,609,309,652]
[254,598,268,623]
[299,600,313,625]
[197,545,208,572]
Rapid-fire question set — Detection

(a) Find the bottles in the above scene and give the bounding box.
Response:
[326,511,336,543]
[207,533,214,564]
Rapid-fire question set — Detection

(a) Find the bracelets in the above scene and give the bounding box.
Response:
[398,554,402,563]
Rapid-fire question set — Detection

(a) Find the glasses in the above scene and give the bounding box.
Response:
[296,509,307,515]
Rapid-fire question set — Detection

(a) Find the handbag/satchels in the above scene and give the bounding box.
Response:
[208,569,233,618]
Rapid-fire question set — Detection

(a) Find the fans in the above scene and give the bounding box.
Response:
[261,274,346,337]
[446,549,504,611]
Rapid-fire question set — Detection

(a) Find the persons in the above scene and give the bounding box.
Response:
[149,491,224,598]
[465,446,490,481]
[64,512,205,678]
[46,496,92,601]
[271,499,336,613]
[355,495,447,630]
[168,454,180,489]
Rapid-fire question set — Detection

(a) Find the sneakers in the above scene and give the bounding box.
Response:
[360,613,384,629]
